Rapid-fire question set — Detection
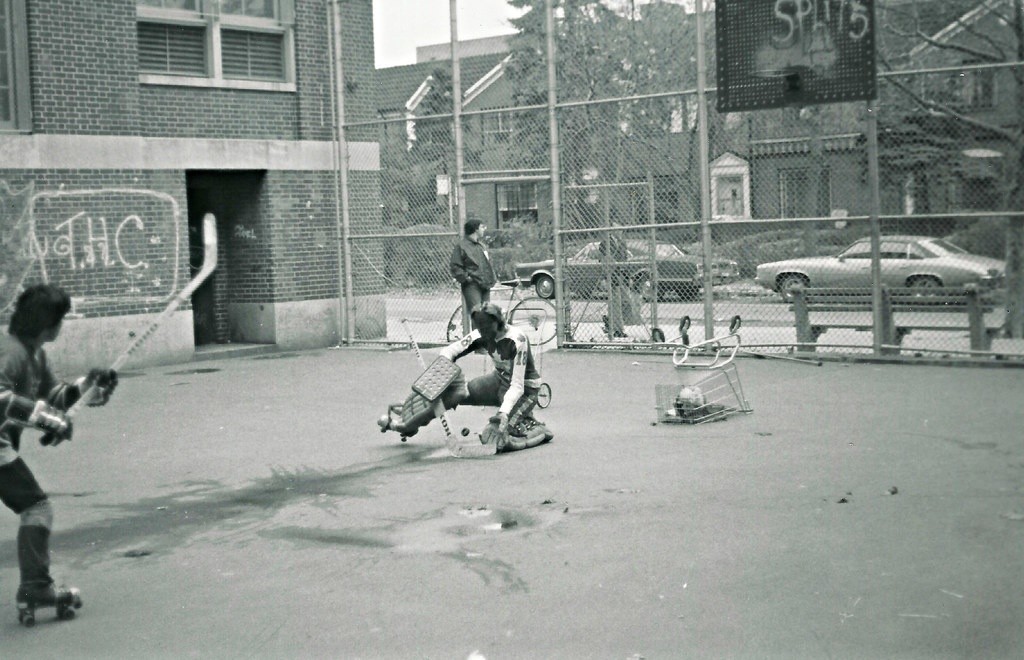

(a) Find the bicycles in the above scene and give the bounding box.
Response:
[446,278,556,345]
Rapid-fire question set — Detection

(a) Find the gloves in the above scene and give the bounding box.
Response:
[28,400,73,446]
[76,367,118,410]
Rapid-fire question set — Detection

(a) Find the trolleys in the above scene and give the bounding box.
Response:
[655,315,754,426]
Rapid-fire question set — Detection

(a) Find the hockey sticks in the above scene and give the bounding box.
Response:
[36,211,220,447]
[401,318,498,459]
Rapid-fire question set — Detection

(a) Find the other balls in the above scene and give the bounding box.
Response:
[460,428,470,436]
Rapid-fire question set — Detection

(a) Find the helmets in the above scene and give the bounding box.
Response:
[673,385,705,417]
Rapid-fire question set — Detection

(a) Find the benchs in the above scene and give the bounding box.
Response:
[787,284,1005,355]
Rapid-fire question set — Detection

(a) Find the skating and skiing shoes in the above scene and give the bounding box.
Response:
[378,416,417,442]
[17,580,82,628]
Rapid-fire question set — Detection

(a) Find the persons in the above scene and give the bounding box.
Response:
[0,285,118,627]
[376,219,635,450]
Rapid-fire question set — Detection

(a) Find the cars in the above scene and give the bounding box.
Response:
[513,238,741,302]
[754,234,1006,307]
